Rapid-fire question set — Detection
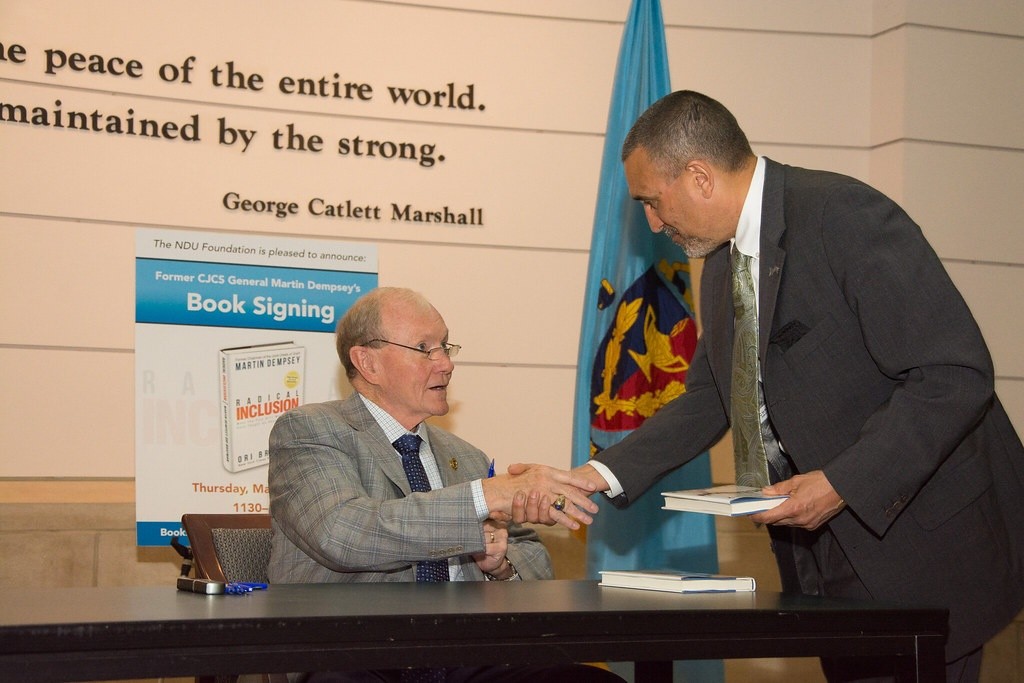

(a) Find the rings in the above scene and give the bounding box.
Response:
[553,495,565,510]
[490,532,495,543]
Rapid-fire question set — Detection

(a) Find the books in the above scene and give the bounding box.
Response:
[220,342,306,473]
[598,569,756,593]
[661,485,790,517]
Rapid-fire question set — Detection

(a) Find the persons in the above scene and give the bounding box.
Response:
[269,288,629,683]
[489,92,1024,683]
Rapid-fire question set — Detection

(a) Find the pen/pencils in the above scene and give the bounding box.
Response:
[225,581,267,595]
[487,458,495,478]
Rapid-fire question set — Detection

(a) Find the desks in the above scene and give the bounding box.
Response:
[0,580,949,683]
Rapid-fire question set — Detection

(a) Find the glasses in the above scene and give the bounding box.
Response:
[358,336,461,362]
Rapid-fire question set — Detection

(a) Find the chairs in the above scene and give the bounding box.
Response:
[182,514,290,683]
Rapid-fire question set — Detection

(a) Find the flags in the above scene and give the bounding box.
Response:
[571,0,727,683]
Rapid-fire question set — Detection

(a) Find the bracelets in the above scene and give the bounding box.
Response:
[486,560,516,582]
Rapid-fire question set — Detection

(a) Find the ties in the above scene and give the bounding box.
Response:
[728,242,771,531]
[390,433,452,585]
